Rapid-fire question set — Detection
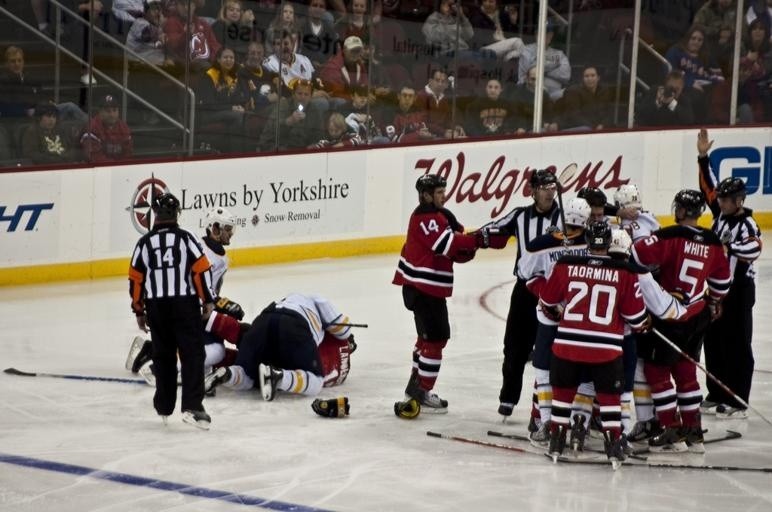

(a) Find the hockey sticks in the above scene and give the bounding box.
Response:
[556,454,771,473]
[555,164,579,248]
[489,430,683,460]
[426,431,601,463]
[633,430,742,456]
[3,367,146,383]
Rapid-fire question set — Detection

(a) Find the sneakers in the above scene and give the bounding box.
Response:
[125,336,282,423]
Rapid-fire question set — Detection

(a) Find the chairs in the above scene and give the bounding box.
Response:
[596,0,657,60]
[1,120,86,165]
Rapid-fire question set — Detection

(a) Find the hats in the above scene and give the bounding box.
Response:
[344,36,363,51]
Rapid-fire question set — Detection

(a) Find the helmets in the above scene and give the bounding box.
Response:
[154,194,182,220]
[206,207,235,237]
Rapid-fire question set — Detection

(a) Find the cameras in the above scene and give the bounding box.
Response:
[664,84,673,97]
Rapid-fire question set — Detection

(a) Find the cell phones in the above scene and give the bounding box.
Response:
[297,100,306,123]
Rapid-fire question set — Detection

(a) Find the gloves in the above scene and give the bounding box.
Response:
[213,296,244,319]
[394,399,420,419]
[312,397,349,418]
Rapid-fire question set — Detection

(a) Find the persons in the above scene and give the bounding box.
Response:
[698,126,763,419]
[391,174,508,413]
[205,293,353,402]
[205,310,356,389]
[480,170,730,461]
[0,0,771,165]
[126,207,235,388]
[129,194,215,424]
